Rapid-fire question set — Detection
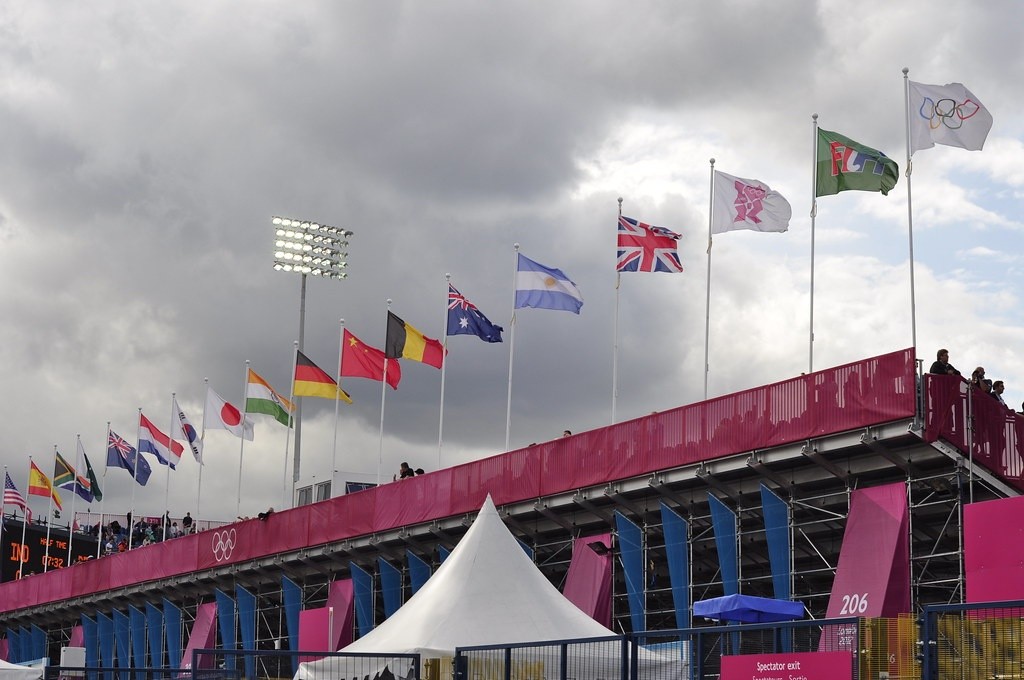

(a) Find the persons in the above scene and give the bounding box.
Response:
[31,506,274,576]
[393,348,1024,481]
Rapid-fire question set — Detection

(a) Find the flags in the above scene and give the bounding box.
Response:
[292,349,352,404]
[513,252,584,315]
[106,428,152,485]
[711,169,792,234]
[618,216,685,272]
[907,79,994,158]
[339,331,401,391]
[138,413,184,470]
[203,383,256,441]
[28,438,103,512]
[3,472,32,525]
[170,396,205,465]
[447,281,504,343]
[384,310,448,369]
[815,127,900,199]
[245,367,295,430]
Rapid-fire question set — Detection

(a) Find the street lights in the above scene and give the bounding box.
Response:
[272,216,354,480]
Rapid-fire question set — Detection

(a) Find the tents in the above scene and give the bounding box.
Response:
[293,494,687,680]
[0,659,42,680]
